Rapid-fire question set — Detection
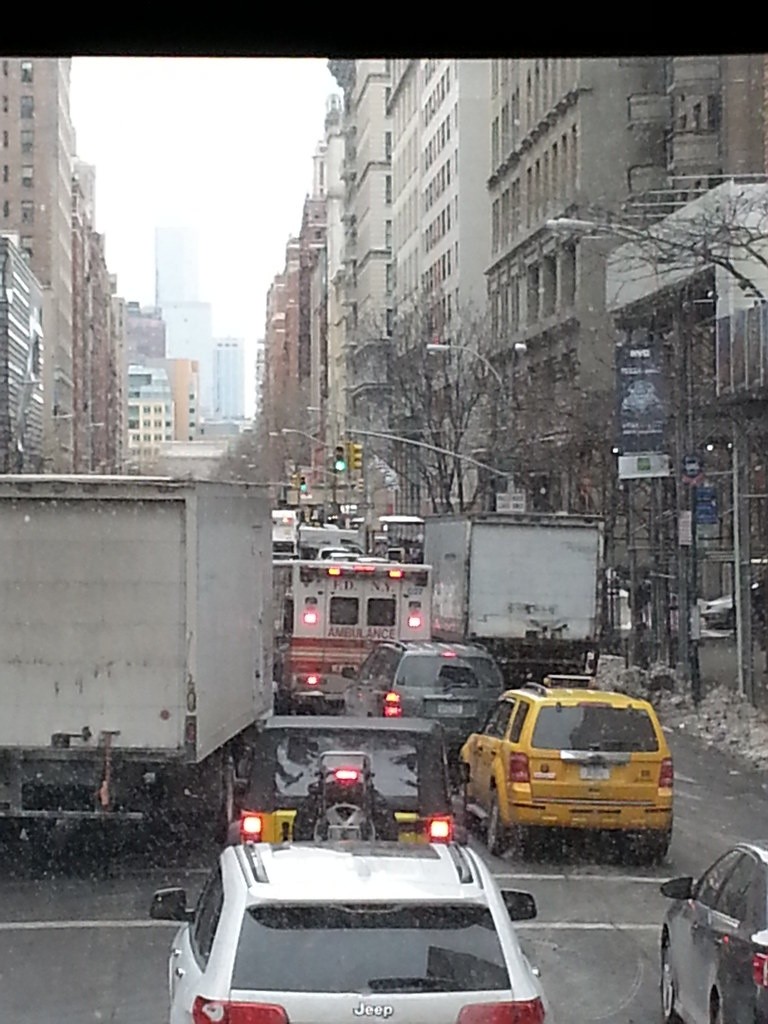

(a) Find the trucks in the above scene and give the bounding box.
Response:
[0,467,279,867]
[417,512,610,690]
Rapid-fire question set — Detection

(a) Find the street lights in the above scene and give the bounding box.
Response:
[424,342,504,387]
[542,215,766,308]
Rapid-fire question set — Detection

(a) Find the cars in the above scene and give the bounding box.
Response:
[234,710,455,851]
[456,678,676,871]
[341,640,510,742]
[262,506,435,709]
[657,835,767,1024]
[148,844,559,1024]
